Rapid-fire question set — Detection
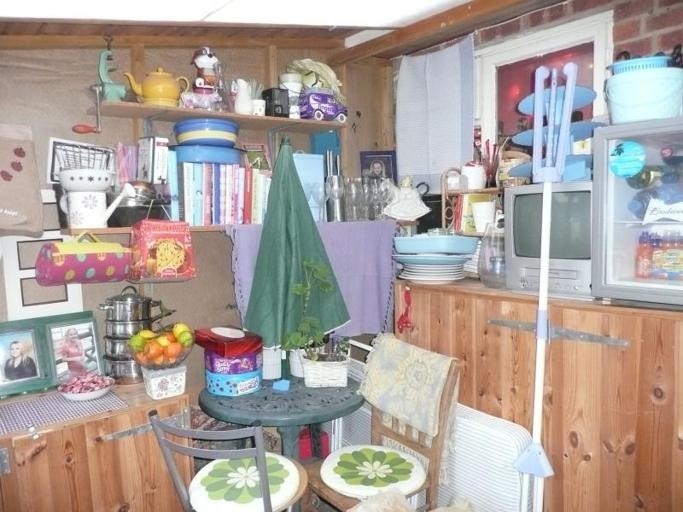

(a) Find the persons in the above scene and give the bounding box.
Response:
[56,326,92,374]
[4,340,37,379]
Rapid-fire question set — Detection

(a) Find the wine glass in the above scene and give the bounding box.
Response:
[301,174,390,222]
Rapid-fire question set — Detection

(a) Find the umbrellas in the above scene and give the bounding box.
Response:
[244,136,351,373]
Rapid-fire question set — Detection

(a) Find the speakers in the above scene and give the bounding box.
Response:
[262,88,290,118]
[417,193,442,234]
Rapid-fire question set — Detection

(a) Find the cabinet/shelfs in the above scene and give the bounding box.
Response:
[60,100,348,236]
[440,167,503,239]
[393,276,683,512]
[0,381,195,512]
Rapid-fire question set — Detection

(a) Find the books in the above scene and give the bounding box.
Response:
[136,136,272,227]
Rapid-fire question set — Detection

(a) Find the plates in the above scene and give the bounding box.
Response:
[390,254,473,284]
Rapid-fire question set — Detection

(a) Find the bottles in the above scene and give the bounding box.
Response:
[632,230,683,284]
[478,223,507,287]
[627,147,682,217]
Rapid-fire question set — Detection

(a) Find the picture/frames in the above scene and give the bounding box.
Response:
[0,327,45,388]
[46,318,103,382]
[359,150,397,188]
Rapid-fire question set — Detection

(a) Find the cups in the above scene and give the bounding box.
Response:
[470,199,495,230]
[427,228,461,237]
[251,100,266,116]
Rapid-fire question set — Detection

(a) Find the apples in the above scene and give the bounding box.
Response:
[128,323,195,368]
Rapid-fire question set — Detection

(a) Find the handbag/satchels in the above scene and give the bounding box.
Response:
[129,219,196,282]
[36,241,130,284]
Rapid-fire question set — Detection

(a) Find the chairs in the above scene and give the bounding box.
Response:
[148,409,309,512]
[300,334,461,512]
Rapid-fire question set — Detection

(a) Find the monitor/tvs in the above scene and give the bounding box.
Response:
[503,180,596,302]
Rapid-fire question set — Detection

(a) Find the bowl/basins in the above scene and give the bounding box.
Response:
[172,118,242,148]
[171,143,250,165]
[109,205,169,225]
[127,335,193,370]
[56,377,114,401]
[53,169,115,192]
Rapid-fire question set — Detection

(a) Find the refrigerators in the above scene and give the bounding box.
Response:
[588,115,683,314]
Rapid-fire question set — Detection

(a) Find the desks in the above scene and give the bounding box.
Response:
[199,375,365,458]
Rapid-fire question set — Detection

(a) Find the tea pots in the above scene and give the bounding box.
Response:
[122,66,189,106]
[234,78,251,114]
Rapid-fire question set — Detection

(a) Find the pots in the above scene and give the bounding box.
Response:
[100,287,175,384]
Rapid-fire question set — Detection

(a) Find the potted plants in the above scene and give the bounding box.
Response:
[283,258,332,378]
[301,331,349,389]
